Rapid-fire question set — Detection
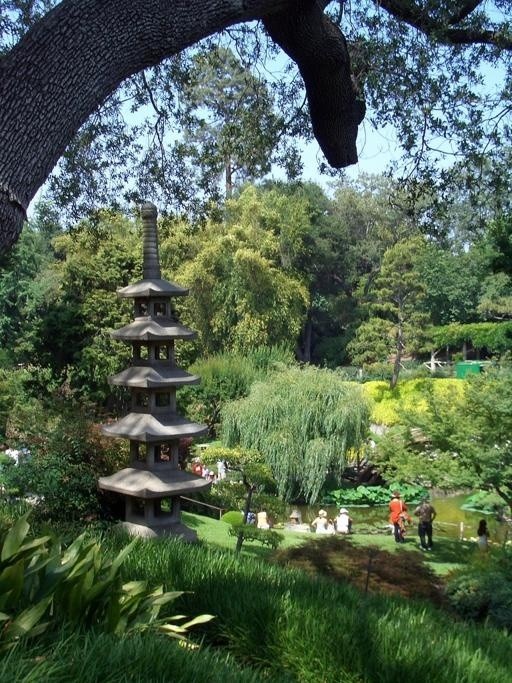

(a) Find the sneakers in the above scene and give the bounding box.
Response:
[418,545,432,551]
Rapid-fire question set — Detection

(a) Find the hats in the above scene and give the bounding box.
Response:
[339,508,348,514]
[319,509,327,517]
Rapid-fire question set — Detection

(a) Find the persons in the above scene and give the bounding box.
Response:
[414,495,437,552]
[477,519,490,554]
[388,489,408,542]
[326,518,335,536]
[309,509,328,535]
[335,508,355,536]
[191,455,229,487]
